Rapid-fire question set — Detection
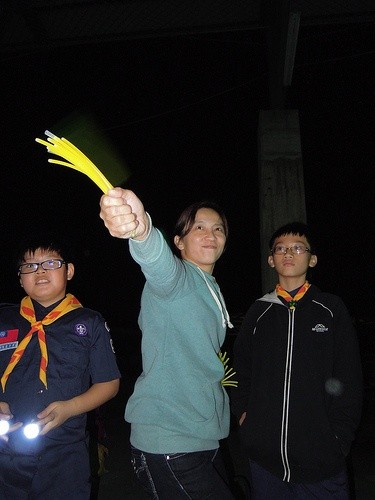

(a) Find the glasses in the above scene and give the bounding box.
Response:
[270,243,311,257]
[16,258,66,273]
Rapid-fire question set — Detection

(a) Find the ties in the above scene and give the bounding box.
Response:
[1,293,83,395]
[274,280,311,311]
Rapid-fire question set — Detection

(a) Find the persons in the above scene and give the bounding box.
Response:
[0,237,121,500]
[229,222,363,500]
[98,187,237,500]
